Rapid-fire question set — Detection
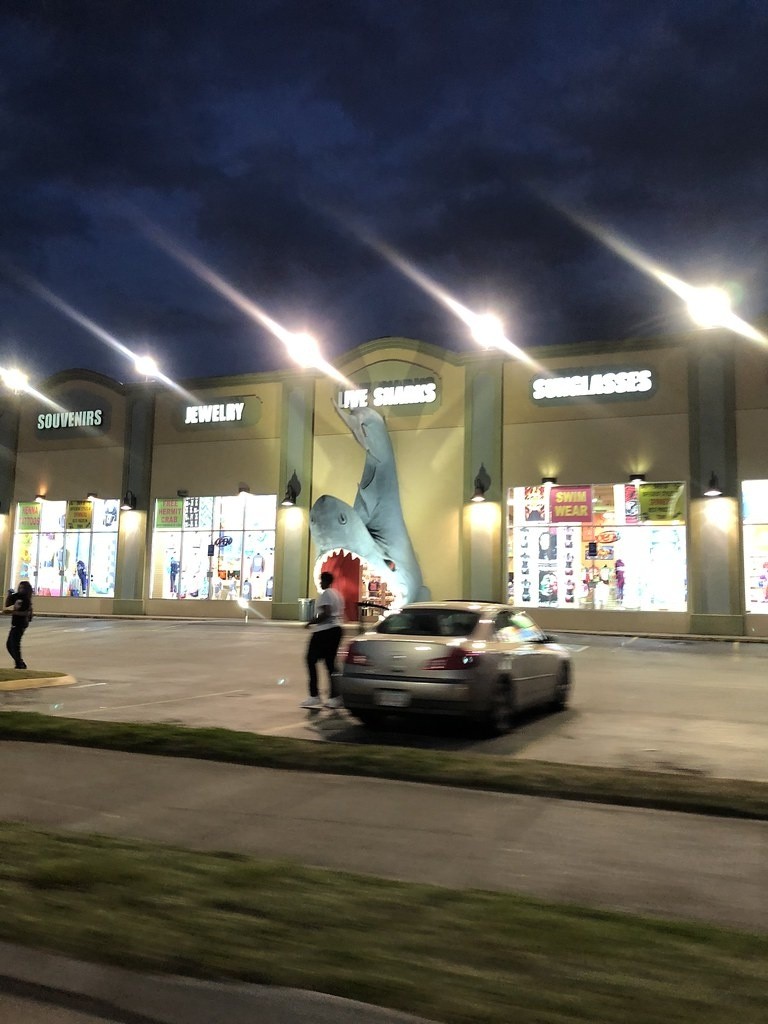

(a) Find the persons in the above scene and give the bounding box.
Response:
[4,580,34,670]
[300,571,349,708]
[583,558,626,605]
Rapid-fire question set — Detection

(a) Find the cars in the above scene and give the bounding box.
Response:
[331,601,573,737]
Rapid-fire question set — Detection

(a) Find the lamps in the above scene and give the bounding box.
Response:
[629,474,645,481]
[470,479,486,502]
[541,478,557,484]
[702,468,723,496]
[281,485,297,506]
[120,490,136,510]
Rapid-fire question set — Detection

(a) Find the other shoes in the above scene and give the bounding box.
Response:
[324,695,345,709]
[300,697,323,708]
